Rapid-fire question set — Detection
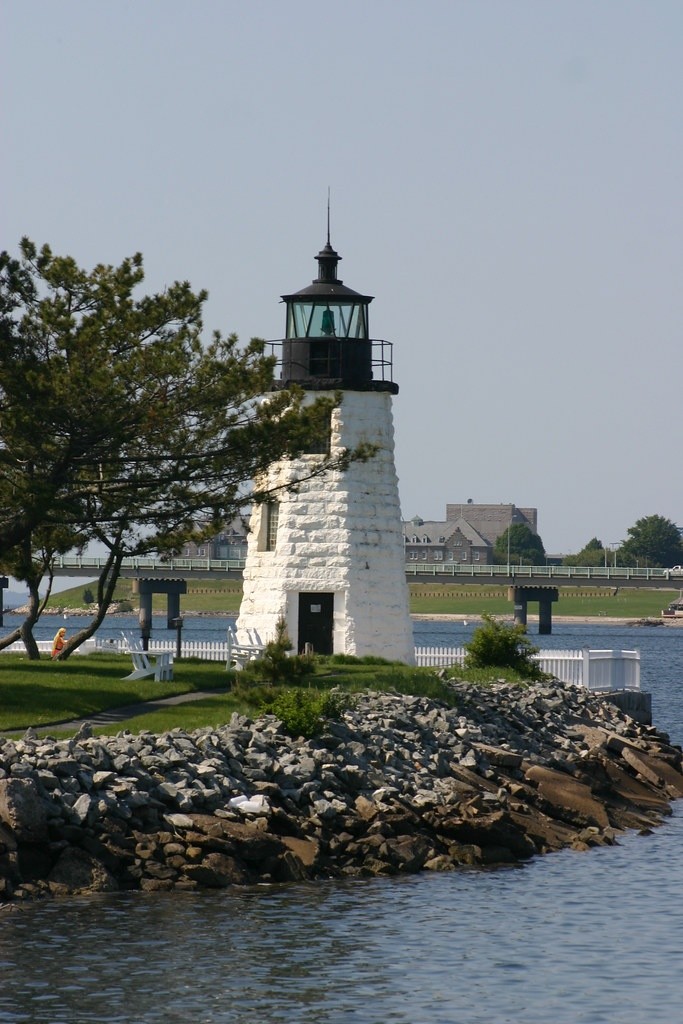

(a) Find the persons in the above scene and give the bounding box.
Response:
[50,628,67,656]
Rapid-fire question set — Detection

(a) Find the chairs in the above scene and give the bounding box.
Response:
[226,625,269,672]
[120,631,174,683]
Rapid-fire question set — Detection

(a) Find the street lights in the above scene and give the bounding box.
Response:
[508,515,518,578]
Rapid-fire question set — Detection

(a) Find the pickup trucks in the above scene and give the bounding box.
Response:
[663,565,683,577]
